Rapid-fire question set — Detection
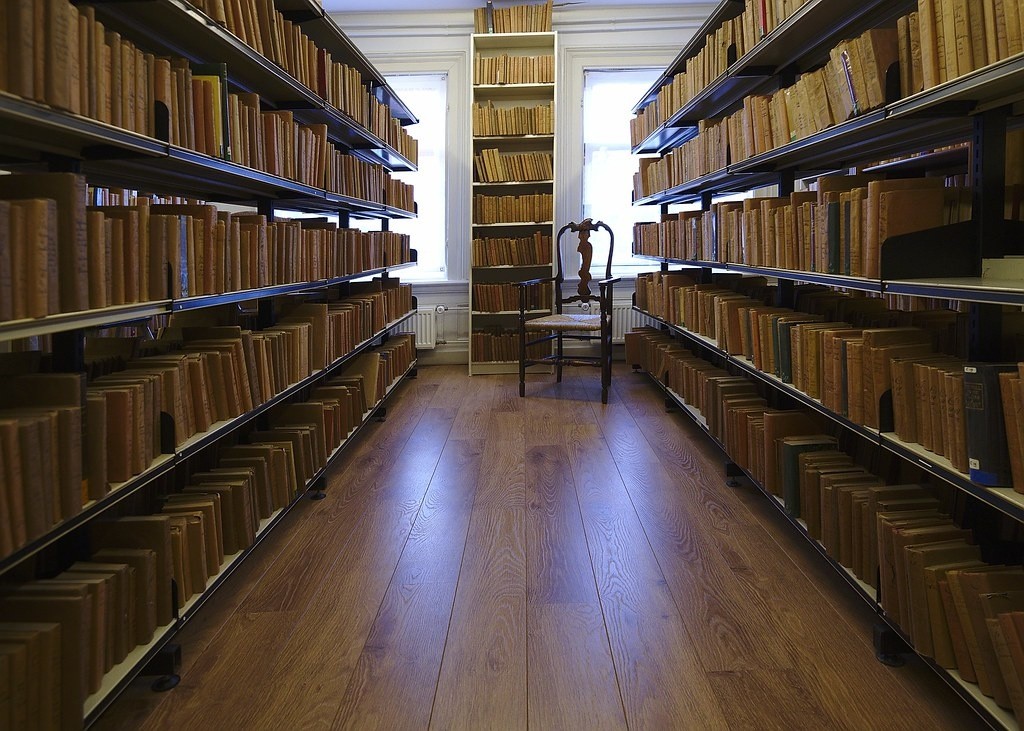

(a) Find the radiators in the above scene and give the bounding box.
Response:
[578,301,663,345]
[386,305,438,350]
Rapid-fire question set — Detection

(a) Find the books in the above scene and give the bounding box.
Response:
[623,0,1024,731]
[0,0,421,731]
[471,0,554,362]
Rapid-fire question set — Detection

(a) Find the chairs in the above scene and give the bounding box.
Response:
[512,218,622,404]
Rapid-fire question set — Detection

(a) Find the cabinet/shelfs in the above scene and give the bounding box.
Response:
[0,0,420,730]
[621,1,1024,731]
[469,31,560,377]
